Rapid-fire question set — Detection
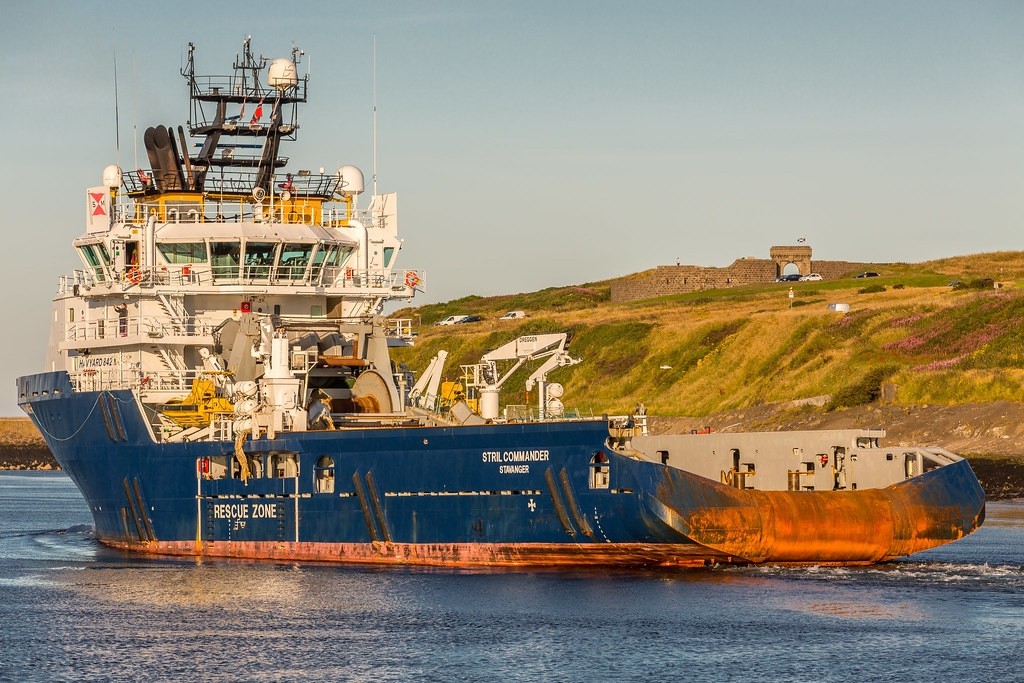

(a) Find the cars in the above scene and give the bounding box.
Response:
[454,316,481,325]
[771,273,823,281]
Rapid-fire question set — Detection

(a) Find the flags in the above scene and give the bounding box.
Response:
[270,99,280,120]
[250,100,263,124]
[240,98,246,119]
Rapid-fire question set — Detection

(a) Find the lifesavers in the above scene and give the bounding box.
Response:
[406,272,419,287]
[127,267,141,283]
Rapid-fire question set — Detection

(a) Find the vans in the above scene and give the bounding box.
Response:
[499,311,525,319]
[434,316,468,326]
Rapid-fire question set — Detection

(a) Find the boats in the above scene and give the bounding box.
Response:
[15,34,986,571]
